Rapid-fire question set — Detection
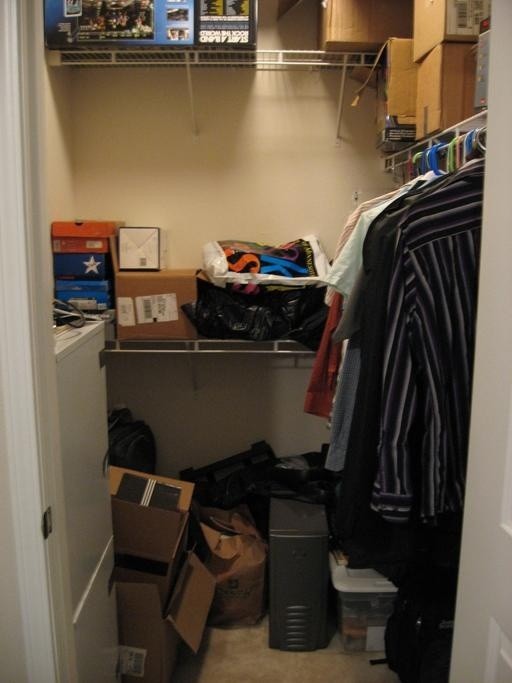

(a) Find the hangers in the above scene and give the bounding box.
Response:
[377,109,484,203]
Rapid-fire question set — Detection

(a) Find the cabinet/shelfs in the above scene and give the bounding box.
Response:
[57,35,384,133]
[93,332,326,400]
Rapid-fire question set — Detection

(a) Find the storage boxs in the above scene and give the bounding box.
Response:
[105,454,248,683]
[49,210,206,339]
[42,1,271,56]
[315,3,487,148]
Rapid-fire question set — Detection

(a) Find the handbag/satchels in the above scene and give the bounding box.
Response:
[189,277,328,340]
[202,234,336,289]
